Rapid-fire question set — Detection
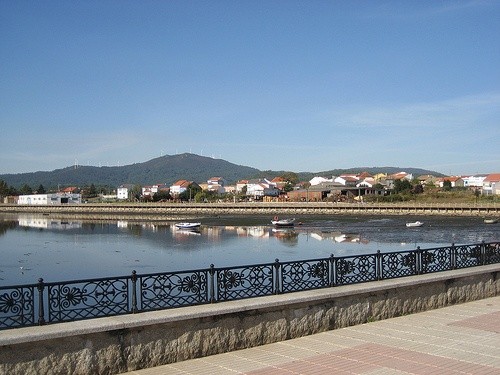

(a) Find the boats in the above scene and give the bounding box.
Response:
[404,221,424,228]
[484,218,499,224]
[174,221,203,229]
[270,216,296,226]
